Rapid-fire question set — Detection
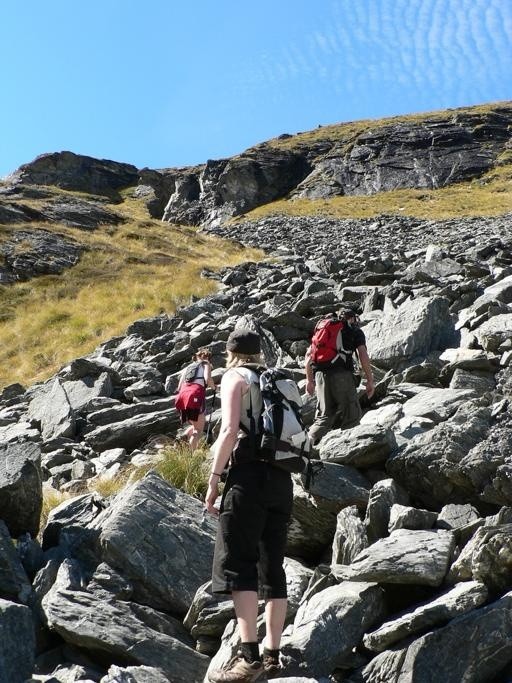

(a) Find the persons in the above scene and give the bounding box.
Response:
[202,329,303,682]
[173,345,218,449]
[305,304,375,444]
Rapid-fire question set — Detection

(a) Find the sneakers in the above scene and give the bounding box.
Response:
[208,652,280,682]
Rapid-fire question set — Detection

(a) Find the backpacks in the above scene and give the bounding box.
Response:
[311,318,353,370]
[239,364,310,471]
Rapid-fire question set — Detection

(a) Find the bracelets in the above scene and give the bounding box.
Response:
[209,468,223,478]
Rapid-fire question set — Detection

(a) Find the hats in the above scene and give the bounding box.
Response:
[226,329,260,353]
[337,308,355,317]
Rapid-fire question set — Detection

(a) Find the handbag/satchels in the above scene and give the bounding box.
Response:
[185,365,198,380]
